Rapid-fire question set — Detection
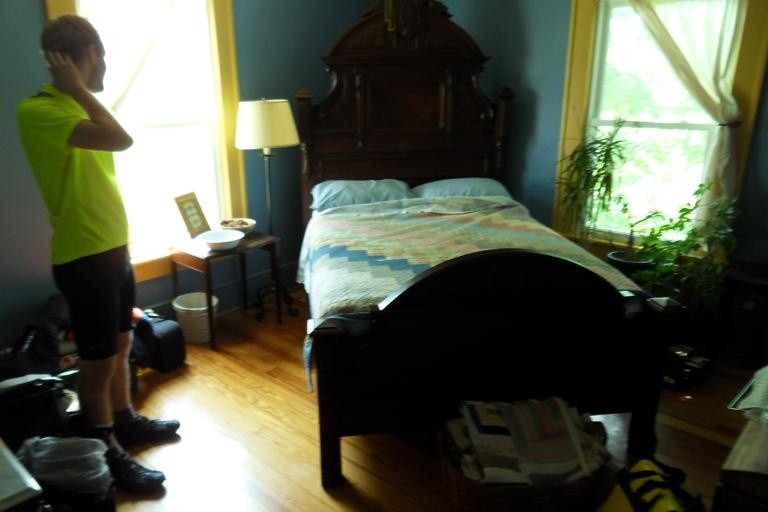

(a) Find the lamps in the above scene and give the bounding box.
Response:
[234,96,302,323]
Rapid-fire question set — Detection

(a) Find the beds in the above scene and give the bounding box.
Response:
[294,3,689,490]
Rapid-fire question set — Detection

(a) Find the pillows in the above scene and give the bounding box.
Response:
[309,175,514,213]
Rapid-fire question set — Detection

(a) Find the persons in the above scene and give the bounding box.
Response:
[11,13,181,494]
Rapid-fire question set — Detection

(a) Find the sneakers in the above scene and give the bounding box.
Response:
[107,452,165,492]
[116,415,180,448]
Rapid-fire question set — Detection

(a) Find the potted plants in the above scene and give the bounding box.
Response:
[551,112,743,296]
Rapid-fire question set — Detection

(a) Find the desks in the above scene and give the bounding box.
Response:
[169,232,284,351]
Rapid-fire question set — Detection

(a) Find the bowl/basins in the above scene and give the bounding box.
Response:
[218,217,257,237]
[204,229,246,251]
